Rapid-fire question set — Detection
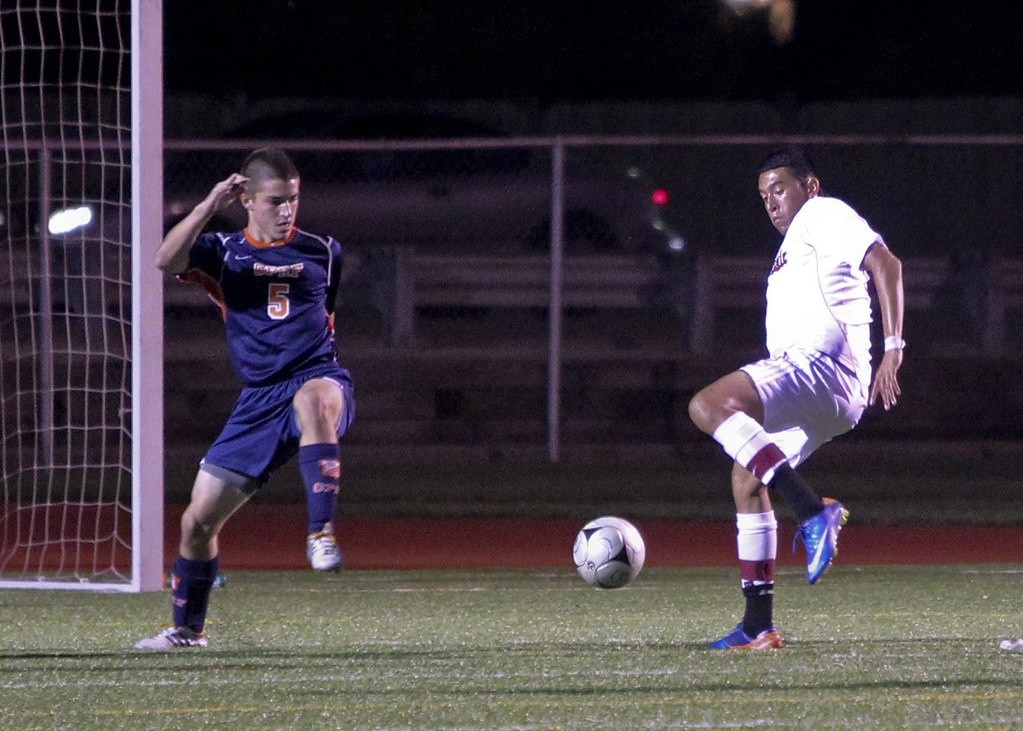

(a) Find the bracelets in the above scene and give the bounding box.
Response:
[883,334,904,352]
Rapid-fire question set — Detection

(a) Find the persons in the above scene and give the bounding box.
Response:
[134,147,353,650]
[688,150,907,653]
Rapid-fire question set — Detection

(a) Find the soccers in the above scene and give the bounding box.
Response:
[572,516,647,590]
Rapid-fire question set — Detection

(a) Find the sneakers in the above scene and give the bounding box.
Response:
[792,497,849,585]
[307,521,342,571]
[708,621,784,651]
[134,626,208,650]
